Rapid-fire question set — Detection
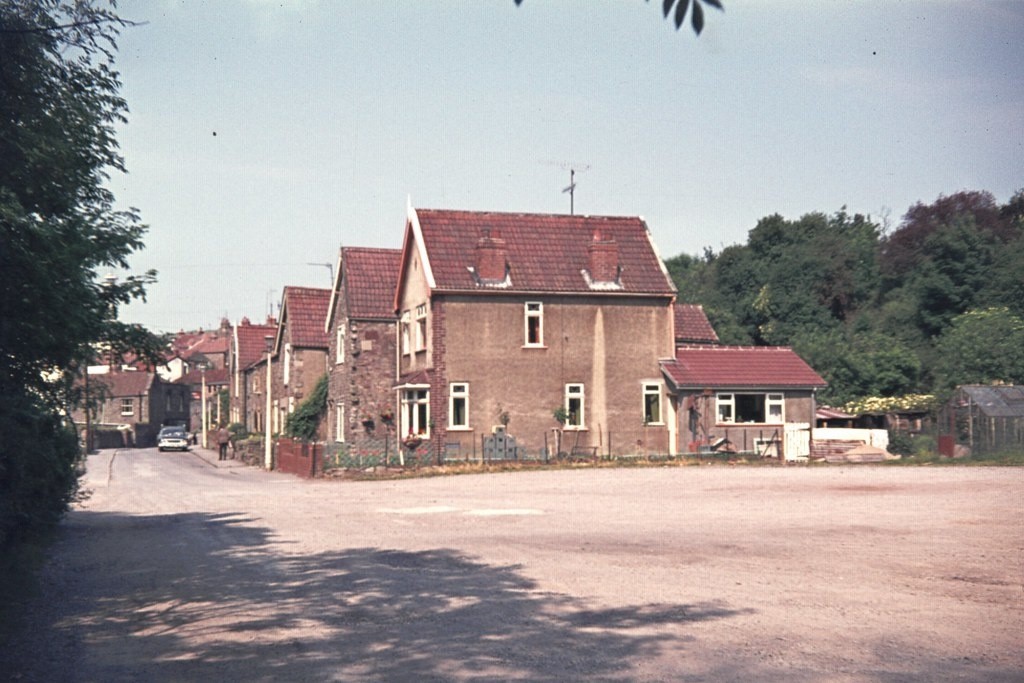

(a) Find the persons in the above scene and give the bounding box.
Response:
[216,425,229,460]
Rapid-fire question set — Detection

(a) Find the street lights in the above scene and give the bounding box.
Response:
[200,365,208,448]
[264,335,275,472]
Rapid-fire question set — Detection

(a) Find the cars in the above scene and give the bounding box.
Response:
[186,432,194,446]
[157,426,188,452]
